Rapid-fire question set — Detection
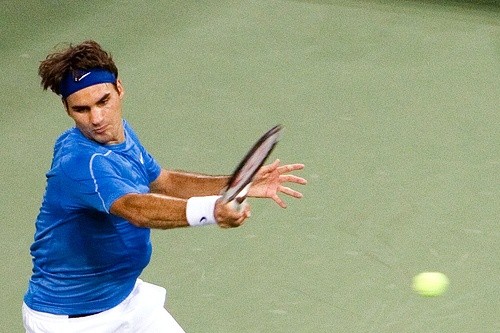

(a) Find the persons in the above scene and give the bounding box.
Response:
[21,37,308,333]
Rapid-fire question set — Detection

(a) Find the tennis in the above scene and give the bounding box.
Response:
[412,271,449,298]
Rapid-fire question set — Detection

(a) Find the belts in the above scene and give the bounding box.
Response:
[68,311,101,318]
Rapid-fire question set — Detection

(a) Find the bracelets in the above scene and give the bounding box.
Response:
[185,194,223,227]
